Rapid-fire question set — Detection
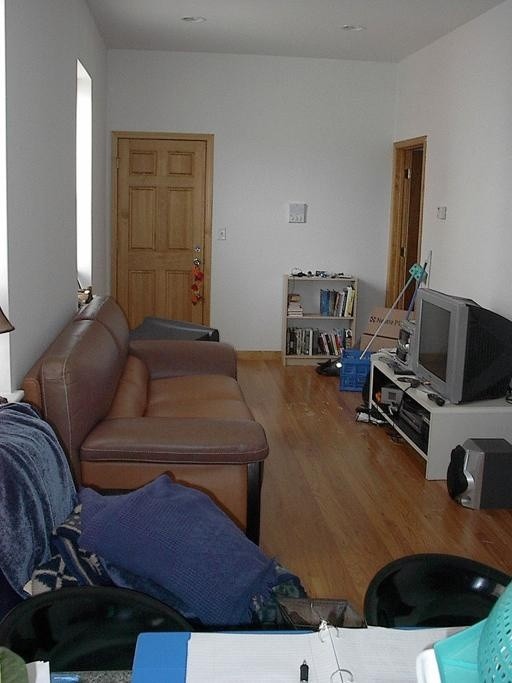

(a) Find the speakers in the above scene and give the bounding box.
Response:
[395,320,415,366]
[446,438,512,511]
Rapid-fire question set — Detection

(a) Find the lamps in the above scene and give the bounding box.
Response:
[0,307,15,334]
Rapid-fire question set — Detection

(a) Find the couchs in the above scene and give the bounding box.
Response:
[23,295,269,546]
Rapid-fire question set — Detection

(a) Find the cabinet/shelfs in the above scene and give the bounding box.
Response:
[283,274,359,366]
[369,349,512,482]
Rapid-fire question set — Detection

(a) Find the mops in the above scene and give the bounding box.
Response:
[358,264,429,360]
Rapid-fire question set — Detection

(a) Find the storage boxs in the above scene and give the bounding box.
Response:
[361,306,416,353]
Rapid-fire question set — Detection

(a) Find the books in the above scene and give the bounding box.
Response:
[320,287,355,317]
[287,294,303,317]
[286,327,352,356]
[185,619,448,683]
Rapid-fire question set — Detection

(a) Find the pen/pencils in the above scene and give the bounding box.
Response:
[300,661,308,683]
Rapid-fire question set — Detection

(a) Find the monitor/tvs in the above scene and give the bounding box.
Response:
[407,288,512,405]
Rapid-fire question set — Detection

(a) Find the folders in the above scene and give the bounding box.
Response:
[131,626,463,683]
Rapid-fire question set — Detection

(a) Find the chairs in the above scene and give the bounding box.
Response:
[0,586,195,672]
[362,553,511,628]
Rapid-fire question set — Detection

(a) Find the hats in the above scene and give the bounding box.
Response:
[1,588,194,671]
